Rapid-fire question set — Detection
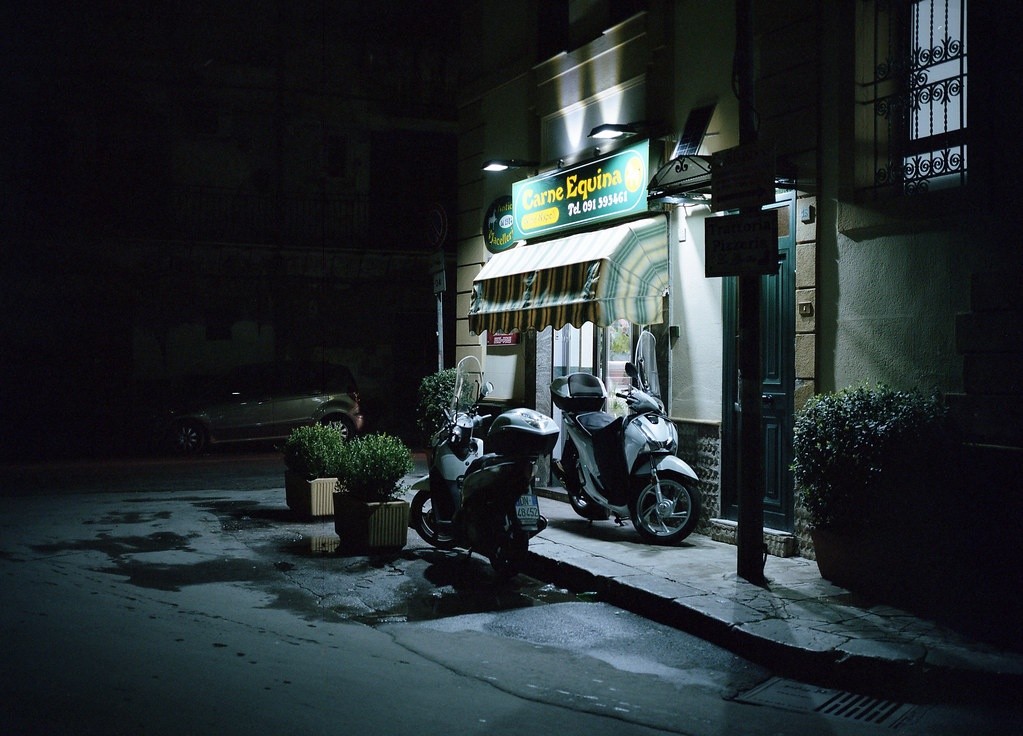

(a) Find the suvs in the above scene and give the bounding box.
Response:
[154,359,367,459]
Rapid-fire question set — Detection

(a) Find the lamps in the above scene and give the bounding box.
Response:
[587,120,671,142]
[481,158,537,172]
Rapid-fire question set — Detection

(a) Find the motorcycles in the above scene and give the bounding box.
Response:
[408,353,561,578]
[547,329,704,547]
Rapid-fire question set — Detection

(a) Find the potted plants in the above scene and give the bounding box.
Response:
[418,367,474,469]
[791,382,946,583]
[332,432,416,550]
[283,421,348,518]
